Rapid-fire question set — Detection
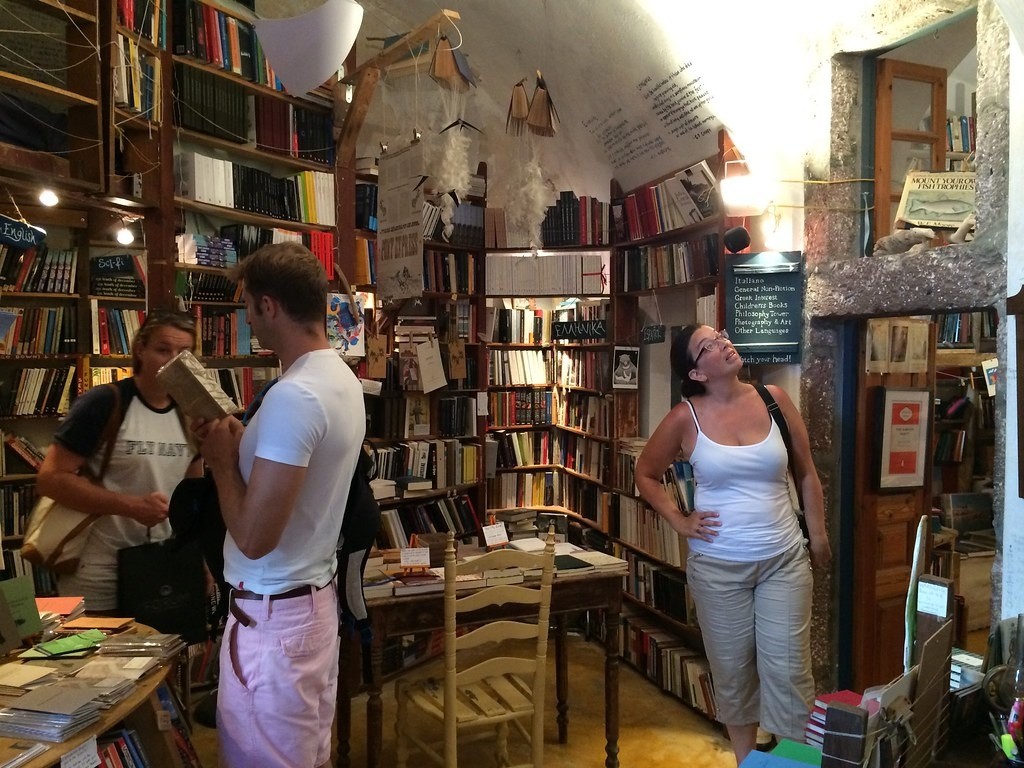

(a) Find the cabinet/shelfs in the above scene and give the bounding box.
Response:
[0,0,996,768]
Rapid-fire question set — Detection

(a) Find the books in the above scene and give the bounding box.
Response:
[950,646,984,692]
[805,106,1023,560]
[738,689,881,768]
[0,0,724,767]
[158,350,237,431]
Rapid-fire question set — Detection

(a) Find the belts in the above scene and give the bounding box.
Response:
[227,584,312,627]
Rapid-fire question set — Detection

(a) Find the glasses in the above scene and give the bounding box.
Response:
[693,329,729,368]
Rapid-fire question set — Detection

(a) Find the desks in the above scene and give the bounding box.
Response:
[337,531,625,768]
[0,616,174,768]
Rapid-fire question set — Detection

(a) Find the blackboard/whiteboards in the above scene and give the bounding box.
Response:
[722,251,804,368]
[551,320,609,340]
[0,213,48,250]
[638,324,667,345]
[92,254,134,274]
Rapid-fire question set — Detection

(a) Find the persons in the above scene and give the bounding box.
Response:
[632,321,833,768]
[189,240,365,767]
[36,310,216,640]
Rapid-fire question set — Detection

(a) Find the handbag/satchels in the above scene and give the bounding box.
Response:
[115,515,210,645]
[20,385,121,575]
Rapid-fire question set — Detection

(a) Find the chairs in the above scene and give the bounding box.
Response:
[395,529,558,768]
